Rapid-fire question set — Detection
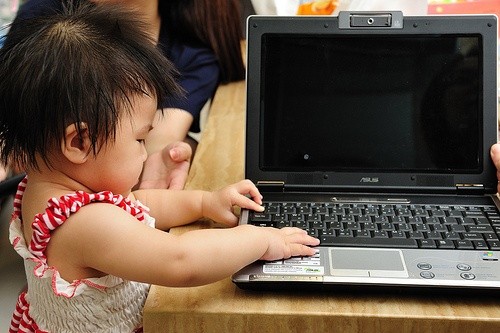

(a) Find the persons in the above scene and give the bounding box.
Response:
[0,0,248,267]
[0,0,320,333]
[490,144,500,195]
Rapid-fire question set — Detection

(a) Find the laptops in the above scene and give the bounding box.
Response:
[231,11,500,294]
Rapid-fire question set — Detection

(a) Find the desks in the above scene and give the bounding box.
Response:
[143,40,500,333]
